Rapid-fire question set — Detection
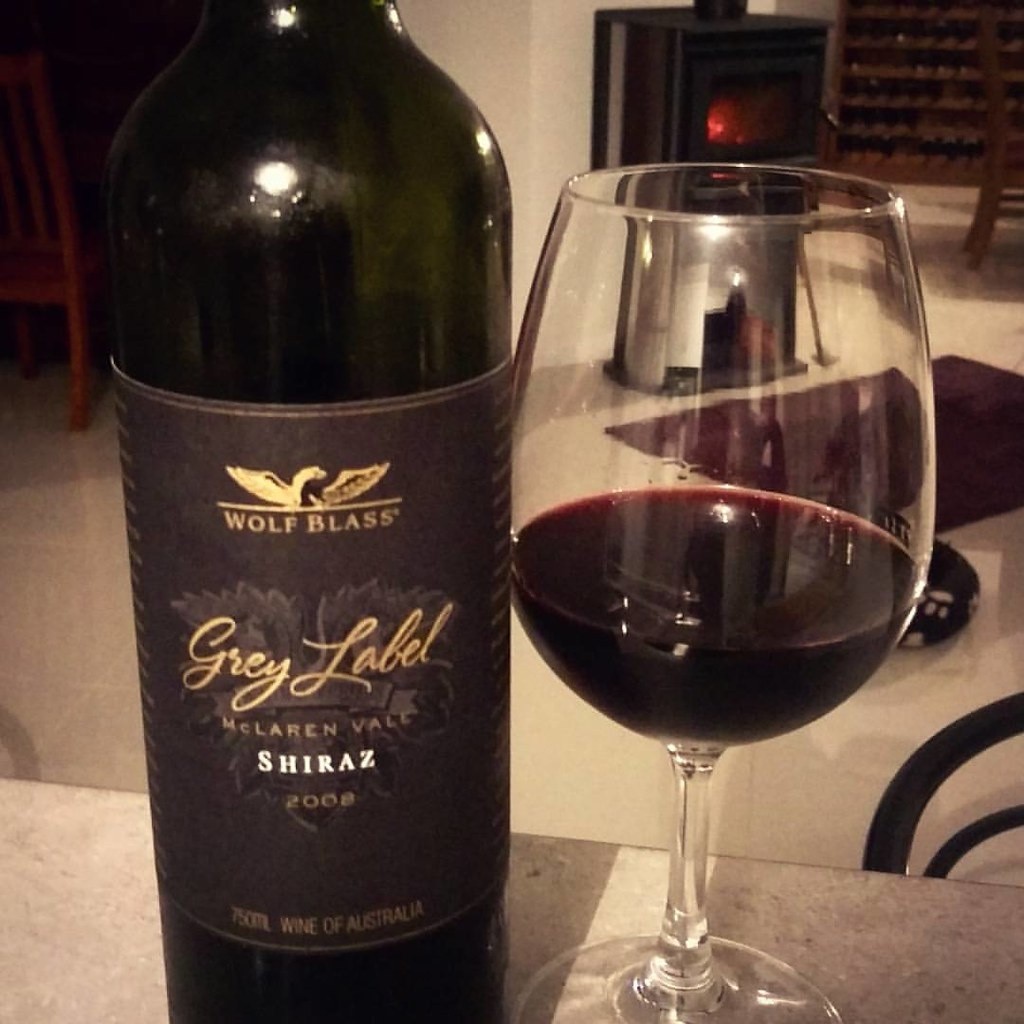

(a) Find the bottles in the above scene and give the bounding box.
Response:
[107,0,512,1024]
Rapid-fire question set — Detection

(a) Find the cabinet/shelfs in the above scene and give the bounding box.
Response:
[814,0,1023,184]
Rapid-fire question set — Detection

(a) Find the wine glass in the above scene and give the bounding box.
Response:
[513,163,936,1022]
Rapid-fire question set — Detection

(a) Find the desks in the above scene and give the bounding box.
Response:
[0,778,1023,1024]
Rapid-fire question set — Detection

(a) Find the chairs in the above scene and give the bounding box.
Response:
[0,51,109,428]
[963,18,1023,272]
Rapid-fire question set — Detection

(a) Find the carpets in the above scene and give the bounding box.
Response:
[605,356,1024,536]
